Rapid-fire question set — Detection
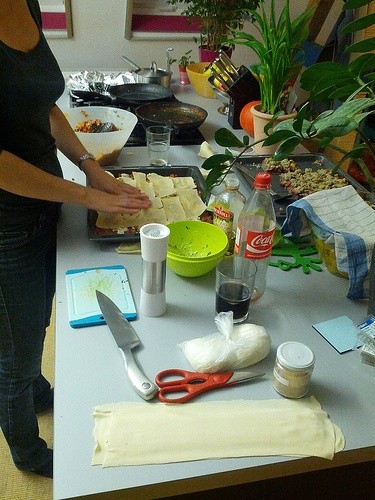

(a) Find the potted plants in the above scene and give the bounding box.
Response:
[169,0,375,204]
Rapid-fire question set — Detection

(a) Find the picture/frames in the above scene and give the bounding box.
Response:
[37,0,73,38]
[125,0,207,40]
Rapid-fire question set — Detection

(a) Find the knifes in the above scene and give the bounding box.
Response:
[208,51,240,90]
[95,290,159,404]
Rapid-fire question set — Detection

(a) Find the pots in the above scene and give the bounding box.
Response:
[121,54,171,86]
[73,83,171,101]
[133,101,208,135]
[69,90,206,147]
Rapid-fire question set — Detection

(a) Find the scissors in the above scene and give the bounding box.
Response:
[155,368,267,403]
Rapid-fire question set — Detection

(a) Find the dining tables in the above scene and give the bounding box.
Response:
[53,72,375,500]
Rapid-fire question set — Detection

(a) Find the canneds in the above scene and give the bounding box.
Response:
[274,341,316,398]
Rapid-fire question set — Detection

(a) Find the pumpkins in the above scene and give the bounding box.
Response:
[240,100,263,138]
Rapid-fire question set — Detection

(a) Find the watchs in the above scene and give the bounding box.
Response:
[78,152,96,171]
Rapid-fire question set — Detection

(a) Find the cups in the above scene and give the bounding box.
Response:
[144,126,170,167]
[215,256,255,324]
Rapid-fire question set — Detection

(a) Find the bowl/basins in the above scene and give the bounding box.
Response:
[64,105,137,167]
[167,220,228,278]
[188,61,237,98]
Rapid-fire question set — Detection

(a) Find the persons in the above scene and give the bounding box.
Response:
[0,0,152,481]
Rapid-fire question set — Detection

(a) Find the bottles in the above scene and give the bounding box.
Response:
[271,341,313,400]
[212,179,246,260]
[234,172,274,301]
[137,224,170,317]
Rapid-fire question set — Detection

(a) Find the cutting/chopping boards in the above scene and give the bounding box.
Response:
[64,264,136,329]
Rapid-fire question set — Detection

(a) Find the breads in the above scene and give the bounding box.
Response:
[260,157,350,200]
